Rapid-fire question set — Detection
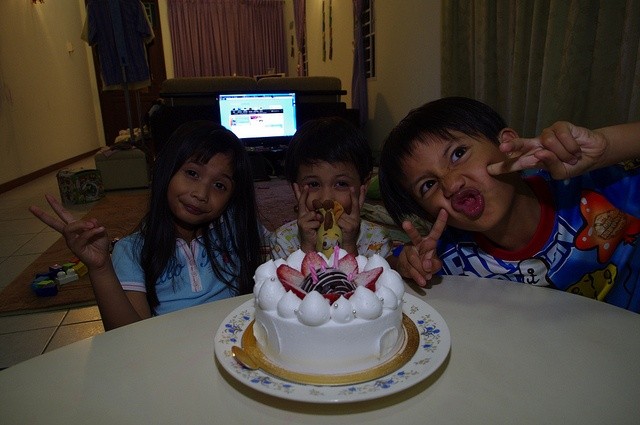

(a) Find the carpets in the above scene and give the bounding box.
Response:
[0,178,405,317]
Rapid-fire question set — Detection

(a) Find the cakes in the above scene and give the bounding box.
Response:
[252,246,405,377]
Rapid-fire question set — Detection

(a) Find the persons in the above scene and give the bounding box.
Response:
[269,116,396,262]
[28,120,270,331]
[378,96,640,318]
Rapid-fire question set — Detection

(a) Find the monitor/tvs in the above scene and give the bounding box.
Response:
[214,90,300,151]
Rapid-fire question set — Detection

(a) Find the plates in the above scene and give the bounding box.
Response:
[214,292,451,404]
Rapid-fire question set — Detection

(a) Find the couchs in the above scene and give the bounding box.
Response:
[94,149,150,190]
[147,76,362,161]
